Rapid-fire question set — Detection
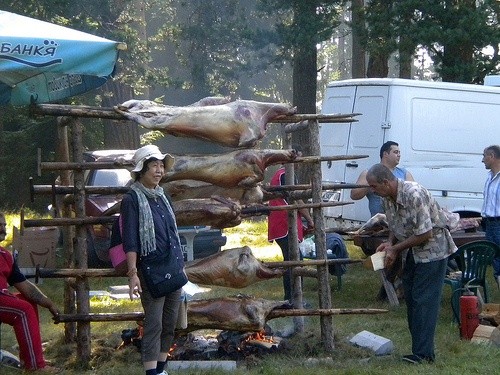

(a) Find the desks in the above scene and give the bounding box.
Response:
[354,231,486,303]
[178,230,200,261]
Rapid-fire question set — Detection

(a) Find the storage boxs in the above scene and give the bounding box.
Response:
[12,226,58,269]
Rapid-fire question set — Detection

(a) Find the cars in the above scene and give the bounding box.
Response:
[54,149,227,271]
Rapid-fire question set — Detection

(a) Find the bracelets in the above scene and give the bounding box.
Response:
[127,268,137,277]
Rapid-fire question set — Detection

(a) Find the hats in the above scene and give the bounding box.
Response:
[129,144,175,180]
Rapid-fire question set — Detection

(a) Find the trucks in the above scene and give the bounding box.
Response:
[320,73,500,270]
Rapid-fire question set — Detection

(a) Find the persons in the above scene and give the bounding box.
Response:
[119,144,186,375]
[365,163,459,364]
[0,211,61,374]
[481,147,500,290]
[350,142,413,300]
[268,144,316,309]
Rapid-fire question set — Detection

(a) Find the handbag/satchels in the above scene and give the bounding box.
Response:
[141,246,189,299]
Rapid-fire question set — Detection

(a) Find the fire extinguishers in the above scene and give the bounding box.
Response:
[450,277,481,341]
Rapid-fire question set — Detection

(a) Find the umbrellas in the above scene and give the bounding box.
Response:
[0,9,127,109]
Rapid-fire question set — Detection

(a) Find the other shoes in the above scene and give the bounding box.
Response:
[43,357,56,366]
[34,364,62,375]
[403,353,434,364]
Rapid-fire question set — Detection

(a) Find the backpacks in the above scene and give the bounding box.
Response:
[108,190,139,275]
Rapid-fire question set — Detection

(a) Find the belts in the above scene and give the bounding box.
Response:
[483,216,500,222]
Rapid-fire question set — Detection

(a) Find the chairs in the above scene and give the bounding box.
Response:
[445,240,500,324]
